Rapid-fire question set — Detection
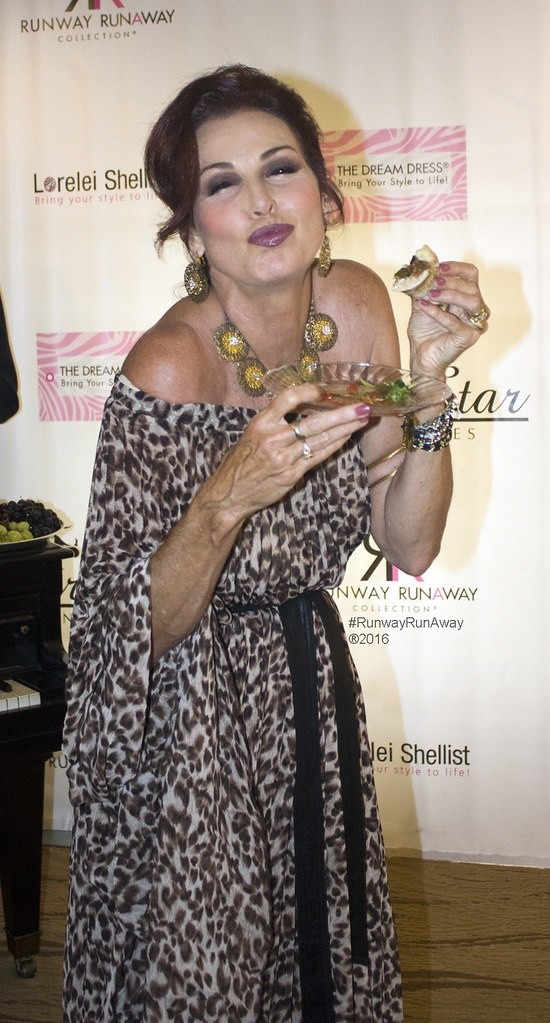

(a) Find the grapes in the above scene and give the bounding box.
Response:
[0,499,62,543]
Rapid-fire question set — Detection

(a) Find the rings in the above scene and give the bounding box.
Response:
[465,303,489,329]
[290,420,314,460]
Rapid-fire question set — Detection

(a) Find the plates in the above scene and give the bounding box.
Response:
[263,361,454,417]
[0,513,71,552]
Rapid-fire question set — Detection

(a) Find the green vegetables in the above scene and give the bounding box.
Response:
[359,378,414,403]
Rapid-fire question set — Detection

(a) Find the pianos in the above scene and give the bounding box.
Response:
[0,526,79,979]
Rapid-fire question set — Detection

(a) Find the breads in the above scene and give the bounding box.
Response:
[391,244,448,306]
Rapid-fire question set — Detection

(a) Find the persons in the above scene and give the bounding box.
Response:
[59,64,489,1023]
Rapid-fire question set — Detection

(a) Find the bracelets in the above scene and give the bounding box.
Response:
[401,400,458,453]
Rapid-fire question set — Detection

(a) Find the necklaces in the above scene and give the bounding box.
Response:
[213,266,338,402]
